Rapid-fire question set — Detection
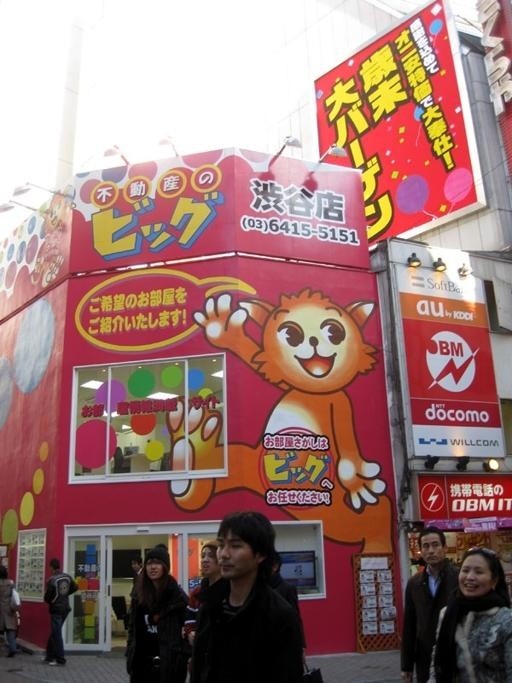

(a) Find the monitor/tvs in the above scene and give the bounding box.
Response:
[74,549,97,575]
[124,446,138,457]
[111,549,140,579]
[277,548,317,588]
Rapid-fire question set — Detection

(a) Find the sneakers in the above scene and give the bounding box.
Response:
[6,649,68,667]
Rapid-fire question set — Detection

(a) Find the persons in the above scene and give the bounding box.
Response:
[426,546,511,682]
[112,446,136,474]
[272,550,307,664]
[0,564,19,657]
[187,509,305,682]
[124,543,190,682]
[181,540,222,682]
[399,525,460,682]
[130,555,143,585]
[39,558,79,666]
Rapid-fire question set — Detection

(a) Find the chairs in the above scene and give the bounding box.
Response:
[111,596,129,620]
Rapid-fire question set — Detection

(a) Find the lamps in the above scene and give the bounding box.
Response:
[103,142,131,166]
[425,455,499,472]
[269,134,303,167]
[318,143,346,163]
[1,199,43,213]
[157,133,180,157]
[408,252,471,276]
[13,179,75,201]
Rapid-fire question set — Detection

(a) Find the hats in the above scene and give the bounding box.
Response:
[145,544,170,566]
[204,538,219,548]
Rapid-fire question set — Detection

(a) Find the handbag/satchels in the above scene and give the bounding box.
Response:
[301,662,323,683]
[10,589,22,611]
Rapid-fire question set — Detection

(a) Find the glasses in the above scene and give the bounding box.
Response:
[463,545,498,558]
